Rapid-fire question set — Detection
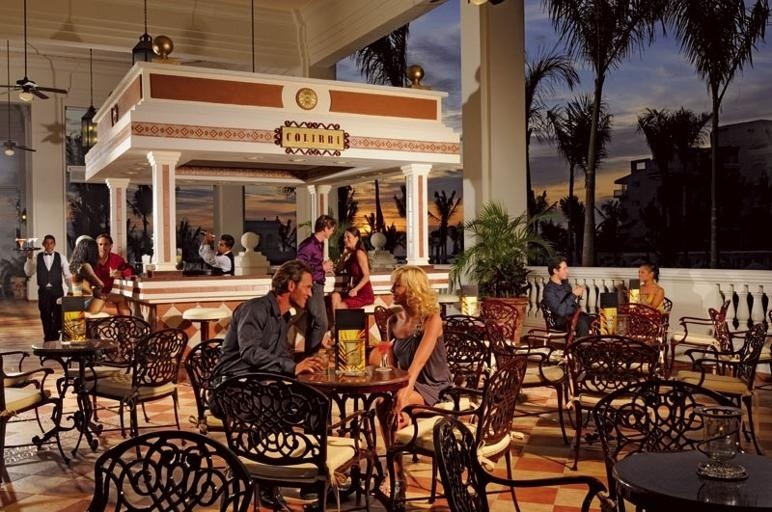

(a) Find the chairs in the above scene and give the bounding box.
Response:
[0,289,772,511]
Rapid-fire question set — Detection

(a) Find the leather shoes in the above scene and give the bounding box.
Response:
[258,482,284,509]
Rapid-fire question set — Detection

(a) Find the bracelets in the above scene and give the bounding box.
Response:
[572,294,576,299]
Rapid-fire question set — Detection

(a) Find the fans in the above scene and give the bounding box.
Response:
[0,0,69,102]
[1,38,37,157]
[427,0,507,6]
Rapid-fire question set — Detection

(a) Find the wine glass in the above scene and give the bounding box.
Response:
[694,404,748,479]
[15,237,39,250]
[698,476,743,506]
[373,340,393,373]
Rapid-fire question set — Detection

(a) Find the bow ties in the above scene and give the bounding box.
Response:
[45,253,52,256]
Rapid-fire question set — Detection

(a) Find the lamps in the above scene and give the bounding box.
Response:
[130,0,156,67]
[469,0,490,7]
[4,147,17,158]
[79,47,101,157]
[20,207,27,226]
[16,90,35,102]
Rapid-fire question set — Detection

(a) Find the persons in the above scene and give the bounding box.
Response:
[199,232,235,275]
[296,215,337,354]
[638,261,665,314]
[375,265,452,511]
[24,234,72,342]
[543,256,591,338]
[210,259,351,503]
[67,238,105,314]
[95,234,135,315]
[330,227,374,338]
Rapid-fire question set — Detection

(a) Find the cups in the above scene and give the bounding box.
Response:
[315,349,330,377]
[176,248,182,262]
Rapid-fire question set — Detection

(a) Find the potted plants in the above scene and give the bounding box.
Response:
[0,255,30,302]
[440,197,573,349]
